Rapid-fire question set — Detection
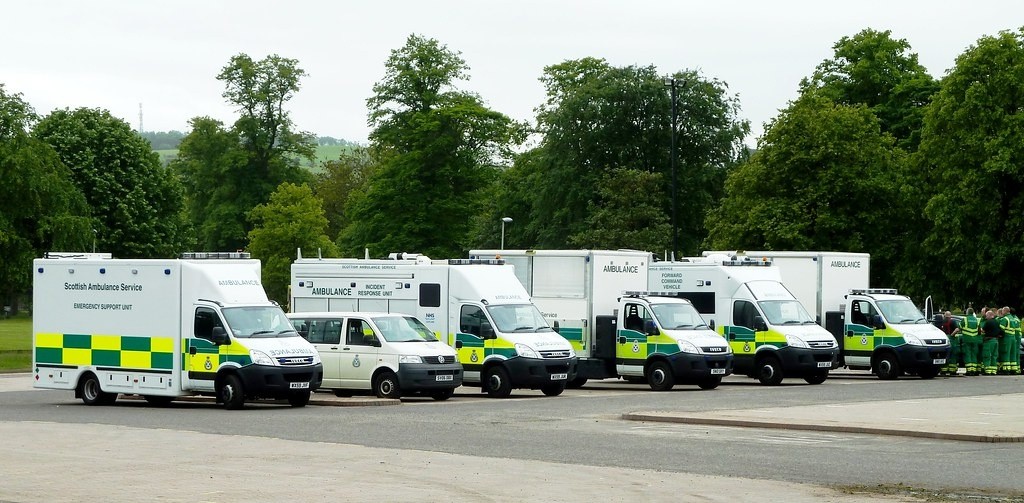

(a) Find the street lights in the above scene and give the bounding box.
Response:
[92,228,98,254]
[500,217,513,250]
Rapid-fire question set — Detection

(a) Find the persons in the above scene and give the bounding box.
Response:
[920,299,931,320]
[980,306,1022,375]
[939,311,959,377]
[981,311,1001,376]
[950,308,981,376]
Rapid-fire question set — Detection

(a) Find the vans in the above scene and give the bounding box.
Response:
[272,311,464,401]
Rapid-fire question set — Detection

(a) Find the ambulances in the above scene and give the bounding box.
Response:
[31,249,323,410]
[701,250,952,381]
[289,247,579,399]
[468,247,735,392]
[649,249,842,386]
